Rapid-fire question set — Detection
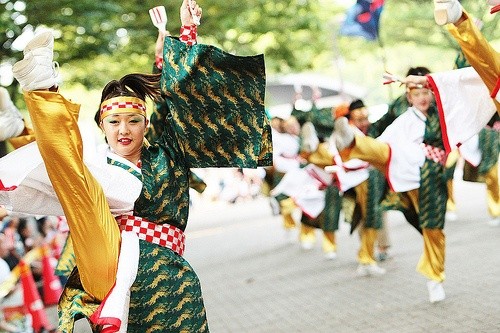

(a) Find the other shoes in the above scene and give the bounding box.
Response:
[324,251,336,259]
[426,279,445,303]
[358,263,386,277]
[335,117,354,151]
[301,122,319,152]
[303,244,314,249]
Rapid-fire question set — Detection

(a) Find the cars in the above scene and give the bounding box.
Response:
[264,74,366,120]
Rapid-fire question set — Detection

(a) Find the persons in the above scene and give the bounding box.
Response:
[0,18,170,312]
[435,0,499,225]
[205,89,410,277]
[335,66,460,303]
[12,1,208,333]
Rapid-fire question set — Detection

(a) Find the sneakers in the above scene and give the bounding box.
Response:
[12,31,61,90]
[434,0,463,25]
[0,87,24,141]
